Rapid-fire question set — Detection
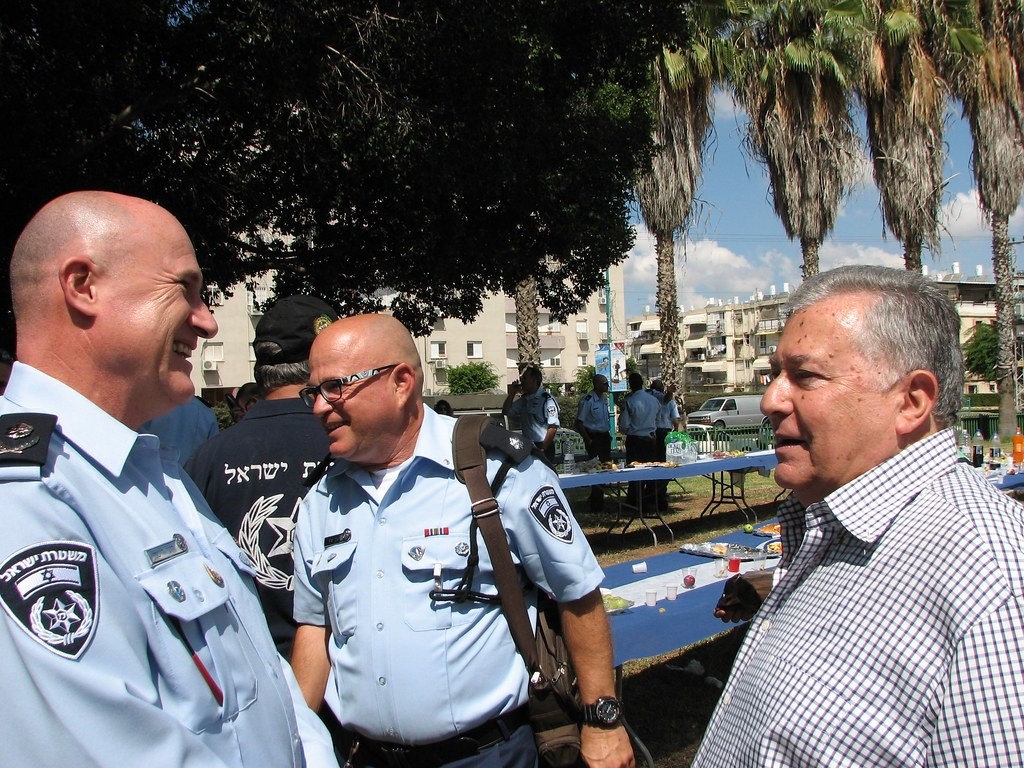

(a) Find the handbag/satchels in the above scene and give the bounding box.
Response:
[529,609,580,768]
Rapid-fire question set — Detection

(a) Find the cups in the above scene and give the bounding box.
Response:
[631,561,648,573]
[646,590,657,606]
[619,462,624,468]
[997,475,1004,484]
[715,552,767,577]
[981,464,990,475]
[667,585,678,600]
[681,567,698,589]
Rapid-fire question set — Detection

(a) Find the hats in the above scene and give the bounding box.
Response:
[253,295,338,365]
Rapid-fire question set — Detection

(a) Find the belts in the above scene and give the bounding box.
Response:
[336,705,529,768]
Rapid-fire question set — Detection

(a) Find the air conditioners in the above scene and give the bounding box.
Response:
[698,354,705,360]
[769,346,777,352]
[775,320,784,327]
[434,308,445,318]
[706,350,715,357]
[578,332,590,340]
[601,332,608,339]
[645,333,652,341]
[698,375,705,381]
[599,297,606,305]
[706,378,714,384]
[203,360,217,371]
[435,360,445,369]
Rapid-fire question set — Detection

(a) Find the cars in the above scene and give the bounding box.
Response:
[685,394,775,455]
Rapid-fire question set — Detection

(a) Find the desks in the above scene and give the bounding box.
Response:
[557,448,1024,768]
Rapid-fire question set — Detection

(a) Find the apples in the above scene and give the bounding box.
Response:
[1009,469,1015,475]
[594,464,602,470]
[744,524,753,533]
[713,452,720,458]
[684,575,695,587]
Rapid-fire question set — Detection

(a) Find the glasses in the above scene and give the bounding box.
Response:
[599,380,608,385]
[299,364,397,407]
[437,407,448,411]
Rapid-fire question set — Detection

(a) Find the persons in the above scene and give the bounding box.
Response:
[188,294,341,663]
[576,374,612,509]
[0,189,340,768]
[618,372,680,507]
[684,265,1024,768]
[284,314,639,768]
[613,360,621,383]
[435,399,453,417]
[502,368,560,463]
[130,394,219,472]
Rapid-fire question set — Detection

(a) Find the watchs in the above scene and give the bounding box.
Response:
[580,695,625,726]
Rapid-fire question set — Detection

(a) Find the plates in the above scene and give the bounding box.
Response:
[756,536,782,557]
[600,587,634,615]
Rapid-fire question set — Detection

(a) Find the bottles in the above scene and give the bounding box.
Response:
[561,431,567,455]
[989,432,1001,470]
[563,438,574,475]
[972,429,983,468]
[958,430,971,462]
[1013,428,1024,467]
